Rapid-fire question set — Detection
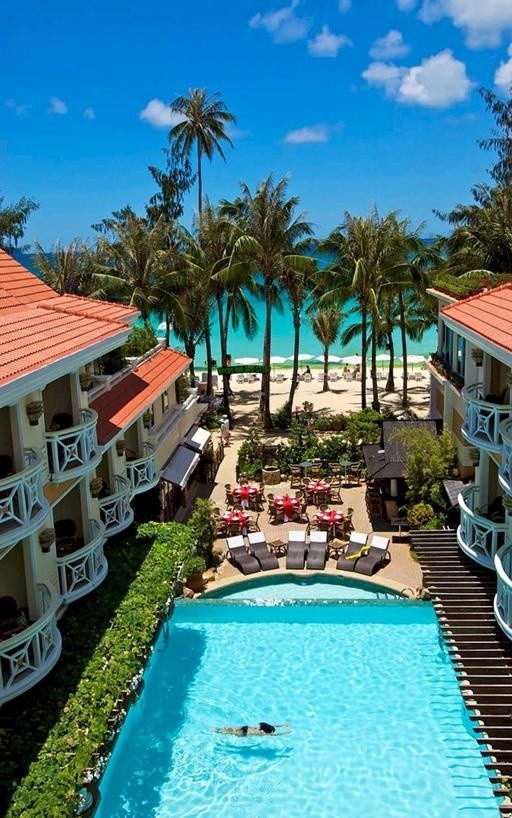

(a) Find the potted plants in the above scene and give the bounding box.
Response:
[318,418,345,431]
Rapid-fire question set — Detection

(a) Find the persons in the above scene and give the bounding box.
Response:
[344,362,350,373]
[237,372,244,379]
[202,720,293,737]
[353,363,361,377]
[219,418,228,446]
[302,364,311,375]
[218,413,231,445]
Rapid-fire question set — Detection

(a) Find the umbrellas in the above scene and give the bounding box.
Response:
[314,353,342,363]
[368,353,398,375]
[233,356,260,372]
[288,352,318,370]
[260,355,287,374]
[157,321,176,333]
[341,354,368,364]
[399,353,425,371]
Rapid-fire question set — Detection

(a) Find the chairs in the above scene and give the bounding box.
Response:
[236,371,422,385]
[213,459,392,577]
[50,412,74,428]
[0,452,13,478]
[0,594,18,637]
[54,518,77,541]
